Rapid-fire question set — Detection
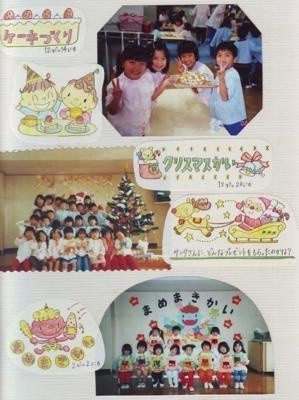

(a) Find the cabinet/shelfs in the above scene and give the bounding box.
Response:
[249,340,274,374]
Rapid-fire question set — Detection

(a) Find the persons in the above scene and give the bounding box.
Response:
[118,325,249,391]
[157,4,261,88]
[175,39,214,104]
[105,45,154,136]
[9,192,140,272]
[207,41,247,134]
[146,41,178,113]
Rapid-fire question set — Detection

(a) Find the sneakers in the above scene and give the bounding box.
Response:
[120,381,244,391]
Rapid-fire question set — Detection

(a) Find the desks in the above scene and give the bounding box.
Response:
[159,31,184,41]
[99,24,159,83]
[143,87,230,136]
[230,33,240,42]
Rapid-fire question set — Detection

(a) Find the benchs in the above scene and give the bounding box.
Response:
[113,374,234,389]
